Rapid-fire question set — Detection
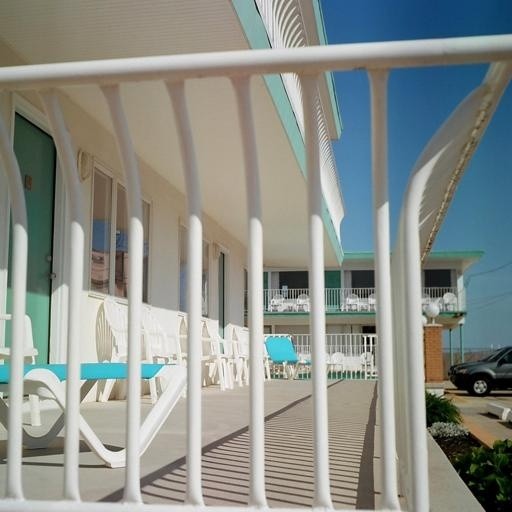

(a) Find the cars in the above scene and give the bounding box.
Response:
[447,345,512,397]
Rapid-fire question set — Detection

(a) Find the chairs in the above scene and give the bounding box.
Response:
[0,314,41,428]
[300,350,374,383]
[264,291,312,315]
[101,295,298,403]
[0,362,189,468]
[340,291,377,314]
[421,291,462,315]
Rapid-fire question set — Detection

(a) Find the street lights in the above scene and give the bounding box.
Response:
[458,317,465,363]
